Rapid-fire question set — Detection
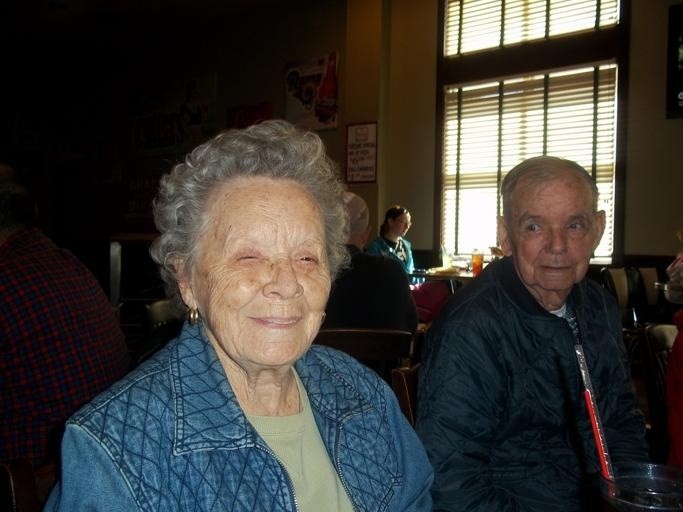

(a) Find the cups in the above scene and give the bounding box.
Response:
[471,253,484,276]
[598,461,683,512]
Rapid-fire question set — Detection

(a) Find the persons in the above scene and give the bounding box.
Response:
[2,157,133,512]
[362,203,433,332]
[40,118,439,512]
[408,154,683,511]
[310,191,420,370]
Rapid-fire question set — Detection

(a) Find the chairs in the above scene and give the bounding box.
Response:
[321,326,418,428]
[638,318,679,461]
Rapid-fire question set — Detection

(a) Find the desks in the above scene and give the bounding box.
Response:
[412,264,477,295]
